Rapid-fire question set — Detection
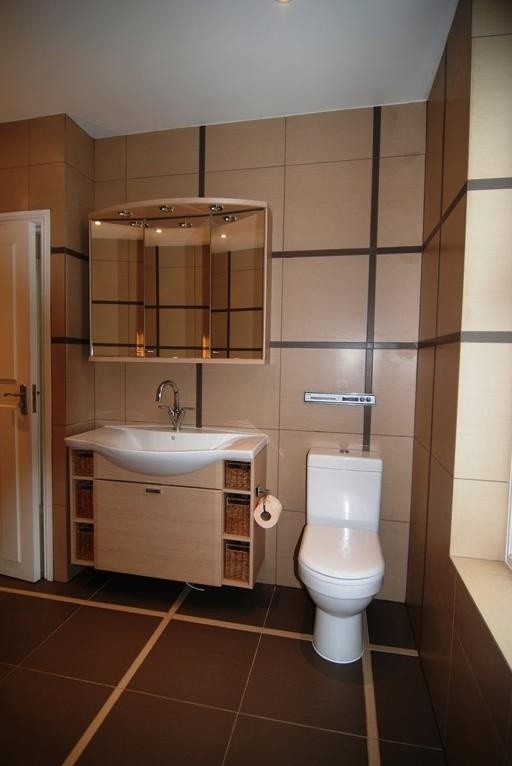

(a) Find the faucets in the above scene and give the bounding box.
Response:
[156,378,179,421]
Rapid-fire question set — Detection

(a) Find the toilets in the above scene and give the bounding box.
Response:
[296,448,384,664]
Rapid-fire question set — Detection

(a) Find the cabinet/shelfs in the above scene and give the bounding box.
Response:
[68,448,266,589]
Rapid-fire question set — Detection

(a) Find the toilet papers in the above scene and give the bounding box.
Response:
[253,495,283,529]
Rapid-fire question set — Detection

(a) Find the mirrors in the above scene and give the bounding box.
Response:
[87,196,272,365]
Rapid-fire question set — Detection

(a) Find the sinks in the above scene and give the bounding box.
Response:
[63,423,268,476]
